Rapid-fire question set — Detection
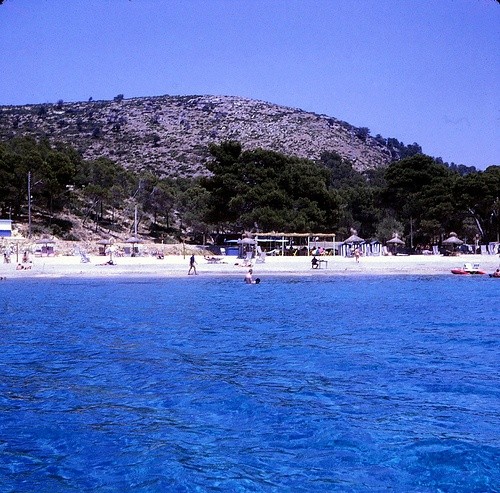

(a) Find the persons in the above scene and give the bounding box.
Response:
[188,253,198,275]
[391,244,496,257]
[4,245,50,270]
[81,245,164,266]
[203,246,363,269]
[494,268,500,277]
[243,269,256,283]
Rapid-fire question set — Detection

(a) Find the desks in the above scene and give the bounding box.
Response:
[313,260,327,269]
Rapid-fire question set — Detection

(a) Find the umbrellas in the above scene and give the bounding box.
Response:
[36,238,56,247]
[386,238,405,246]
[96,239,114,251]
[342,234,364,251]
[237,238,258,252]
[442,236,463,251]
[124,237,142,253]
